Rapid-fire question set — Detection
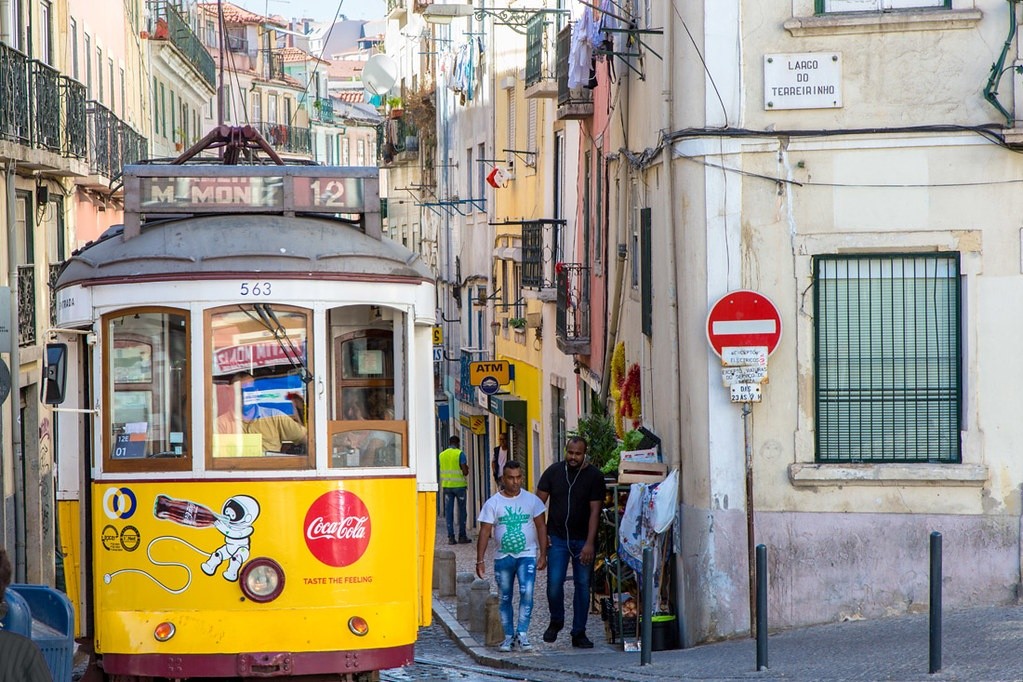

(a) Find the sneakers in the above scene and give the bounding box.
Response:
[448,535,458,545]
[500,637,514,651]
[542,619,563,643]
[457,535,472,542]
[515,634,532,650]
[572,632,595,648]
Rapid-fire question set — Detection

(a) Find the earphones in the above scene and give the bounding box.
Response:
[584,454,586,458]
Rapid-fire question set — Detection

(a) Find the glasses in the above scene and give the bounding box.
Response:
[242,387,258,392]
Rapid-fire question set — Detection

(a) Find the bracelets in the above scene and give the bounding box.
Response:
[475,559,485,567]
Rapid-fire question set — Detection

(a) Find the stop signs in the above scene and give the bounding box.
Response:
[706,290,782,365]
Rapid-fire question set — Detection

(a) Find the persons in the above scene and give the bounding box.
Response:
[212,369,310,457]
[534,437,609,649]
[338,394,397,468]
[437,435,472,544]
[476,459,549,653]
[491,431,511,491]
[1,546,58,682]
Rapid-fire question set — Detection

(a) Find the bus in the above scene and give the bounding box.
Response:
[47,121,433,682]
